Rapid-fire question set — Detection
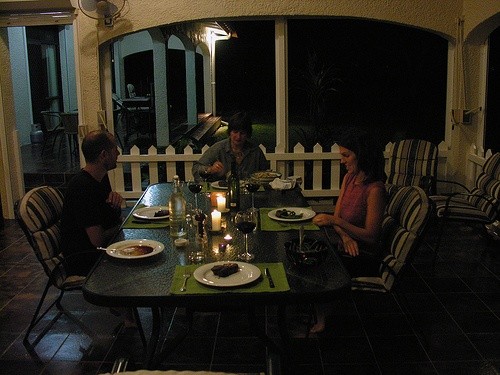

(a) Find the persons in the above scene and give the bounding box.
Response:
[192,118,267,181]
[58,129,149,328]
[311,129,389,335]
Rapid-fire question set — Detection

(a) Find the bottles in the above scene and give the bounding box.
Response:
[168,175,187,240]
[184,209,209,262]
[227,162,240,212]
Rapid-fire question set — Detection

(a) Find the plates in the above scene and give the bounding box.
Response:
[132,206,169,220]
[268,207,316,221]
[211,180,245,189]
[193,260,262,287]
[106,239,164,259]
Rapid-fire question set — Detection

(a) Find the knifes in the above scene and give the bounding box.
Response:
[266,268,275,288]
[132,220,169,224]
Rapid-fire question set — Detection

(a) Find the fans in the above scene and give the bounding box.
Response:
[78,0,126,26]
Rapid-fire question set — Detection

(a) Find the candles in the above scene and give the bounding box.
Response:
[211,209,222,231]
[216,192,227,210]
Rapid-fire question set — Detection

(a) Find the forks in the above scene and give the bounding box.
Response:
[180,274,191,291]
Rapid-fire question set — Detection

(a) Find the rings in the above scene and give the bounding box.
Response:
[317,218,320,221]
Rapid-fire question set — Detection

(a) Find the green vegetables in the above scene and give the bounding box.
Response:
[276,210,304,219]
[288,235,325,252]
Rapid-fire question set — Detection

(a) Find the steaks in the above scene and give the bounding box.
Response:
[276,209,288,217]
[154,210,169,217]
[211,263,238,277]
[218,181,229,187]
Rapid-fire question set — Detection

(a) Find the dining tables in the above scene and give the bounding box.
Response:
[81,182,350,375]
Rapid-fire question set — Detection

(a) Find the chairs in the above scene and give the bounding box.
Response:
[428,152,500,276]
[382,139,439,197]
[36,93,155,159]
[285,186,429,341]
[13,185,146,344]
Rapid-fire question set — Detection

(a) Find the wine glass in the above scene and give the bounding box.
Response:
[235,211,257,260]
[244,177,261,213]
[187,178,203,212]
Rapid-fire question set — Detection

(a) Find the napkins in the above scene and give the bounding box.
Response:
[170,262,290,295]
[121,205,170,229]
[259,207,320,231]
[198,182,265,192]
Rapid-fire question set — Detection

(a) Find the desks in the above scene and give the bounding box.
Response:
[122,97,151,111]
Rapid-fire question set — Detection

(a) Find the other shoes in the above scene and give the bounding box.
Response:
[123,316,150,329]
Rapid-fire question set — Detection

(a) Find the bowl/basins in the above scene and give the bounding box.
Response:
[175,238,188,247]
[251,170,282,182]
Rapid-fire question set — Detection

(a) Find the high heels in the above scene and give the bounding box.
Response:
[310,307,328,333]
